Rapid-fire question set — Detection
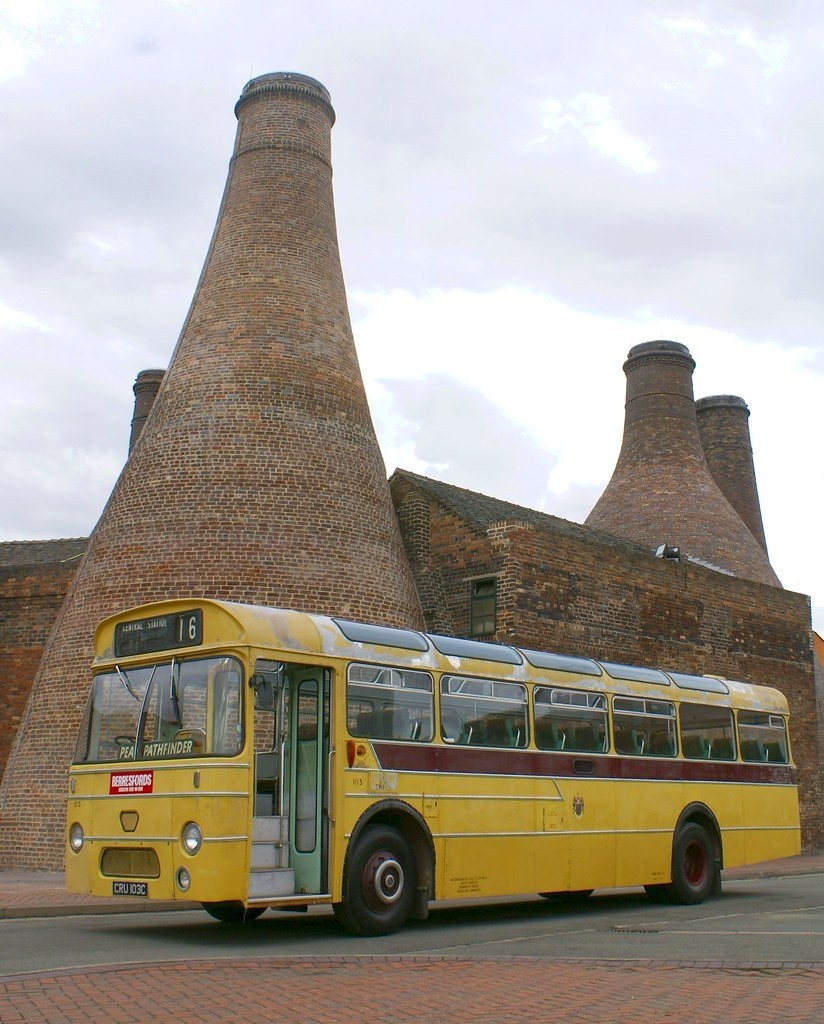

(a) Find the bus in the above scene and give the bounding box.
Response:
[64,597,804,937]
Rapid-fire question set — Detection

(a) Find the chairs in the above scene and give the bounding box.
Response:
[440,716,474,745]
[686,735,712,758]
[412,716,431,741]
[487,718,522,747]
[465,720,489,745]
[537,722,567,750]
[767,743,786,761]
[575,720,606,752]
[381,709,411,738]
[557,721,579,750]
[742,741,770,762]
[354,710,382,738]
[657,734,674,755]
[637,734,649,754]
[616,729,645,754]
[713,738,734,759]
